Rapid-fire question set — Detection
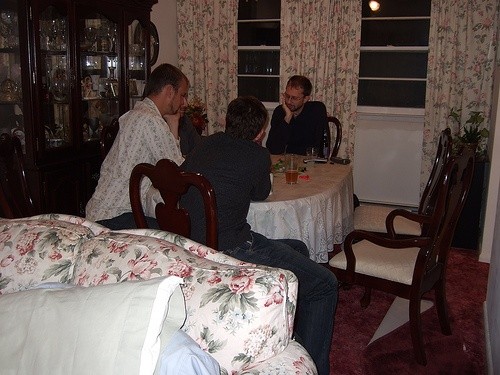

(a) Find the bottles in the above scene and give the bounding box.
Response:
[1,71,22,100]
[106,67,119,96]
[321,129,329,160]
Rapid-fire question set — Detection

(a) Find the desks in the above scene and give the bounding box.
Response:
[446,149,490,251]
[145,154,355,264]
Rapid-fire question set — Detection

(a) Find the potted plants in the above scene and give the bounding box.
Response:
[445,103,490,156]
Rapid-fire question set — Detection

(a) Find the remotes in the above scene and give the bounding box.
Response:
[304,158,327,163]
[331,157,350,164]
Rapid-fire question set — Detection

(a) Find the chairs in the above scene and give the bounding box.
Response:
[0,112,476,366]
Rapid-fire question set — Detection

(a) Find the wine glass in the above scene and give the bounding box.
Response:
[37,17,118,53]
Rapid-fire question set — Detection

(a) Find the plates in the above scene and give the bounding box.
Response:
[52,69,68,100]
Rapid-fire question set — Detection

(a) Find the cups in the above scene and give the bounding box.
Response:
[285,153,299,185]
[306,147,318,160]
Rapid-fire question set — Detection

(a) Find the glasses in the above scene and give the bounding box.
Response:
[282,92,305,101]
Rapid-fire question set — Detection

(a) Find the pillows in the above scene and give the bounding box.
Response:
[29,283,228,375]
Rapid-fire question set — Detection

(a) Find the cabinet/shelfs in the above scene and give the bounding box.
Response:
[0,0,160,219]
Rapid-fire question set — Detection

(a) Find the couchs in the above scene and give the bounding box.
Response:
[0,214,319,375]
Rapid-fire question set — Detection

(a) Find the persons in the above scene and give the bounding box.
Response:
[265,76,329,157]
[85,64,190,230]
[179,96,338,375]
